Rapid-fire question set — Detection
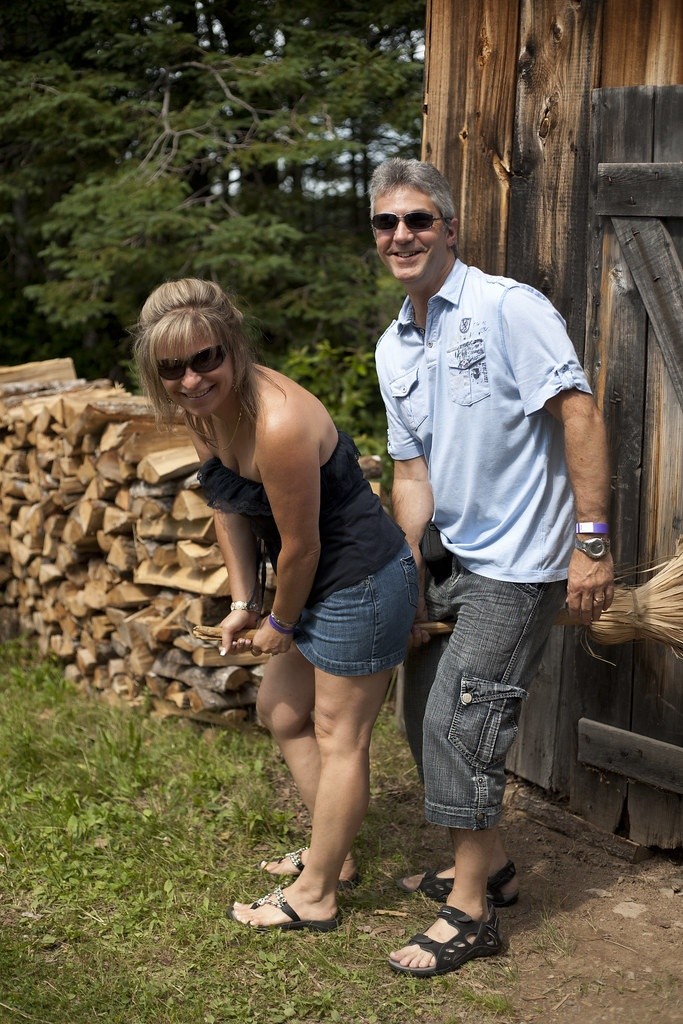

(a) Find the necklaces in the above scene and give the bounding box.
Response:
[202,378,248,449]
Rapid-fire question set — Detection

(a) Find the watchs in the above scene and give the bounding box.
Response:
[574,535,611,559]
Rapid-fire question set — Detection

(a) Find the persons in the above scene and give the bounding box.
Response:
[131,278,418,930]
[366,159,616,976]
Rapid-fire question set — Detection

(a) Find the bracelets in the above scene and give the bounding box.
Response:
[230,601,262,613]
[574,522,610,534]
[270,610,302,627]
[268,615,297,634]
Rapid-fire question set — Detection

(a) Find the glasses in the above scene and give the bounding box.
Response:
[370,211,452,230]
[156,344,229,381]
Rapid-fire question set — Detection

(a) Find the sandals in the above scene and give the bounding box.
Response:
[396,859,520,907]
[388,904,503,978]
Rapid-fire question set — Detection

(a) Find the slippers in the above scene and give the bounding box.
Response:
[225,883,342,933]
[278,846,359,890]
